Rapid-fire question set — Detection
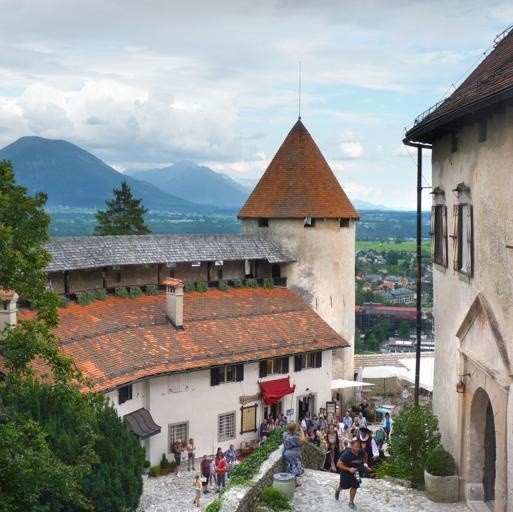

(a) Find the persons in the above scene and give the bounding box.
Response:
[190,444,238,508]
[185,438,196,472]
[255,384,410,477]
[279,422,306,488]
[333,439,370,510]
[172,438,186,477]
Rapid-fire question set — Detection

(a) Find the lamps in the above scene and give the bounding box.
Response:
[455,371,472,395]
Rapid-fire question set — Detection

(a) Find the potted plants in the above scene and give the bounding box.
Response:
[143,452,176,477]
[422,443,459,504]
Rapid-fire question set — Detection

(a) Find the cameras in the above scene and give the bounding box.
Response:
[353,470,362,483]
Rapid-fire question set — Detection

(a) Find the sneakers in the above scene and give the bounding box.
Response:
[349,503,355,509]
[335,490,340,500]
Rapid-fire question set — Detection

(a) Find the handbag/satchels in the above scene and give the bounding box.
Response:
[200,475,208,485]
